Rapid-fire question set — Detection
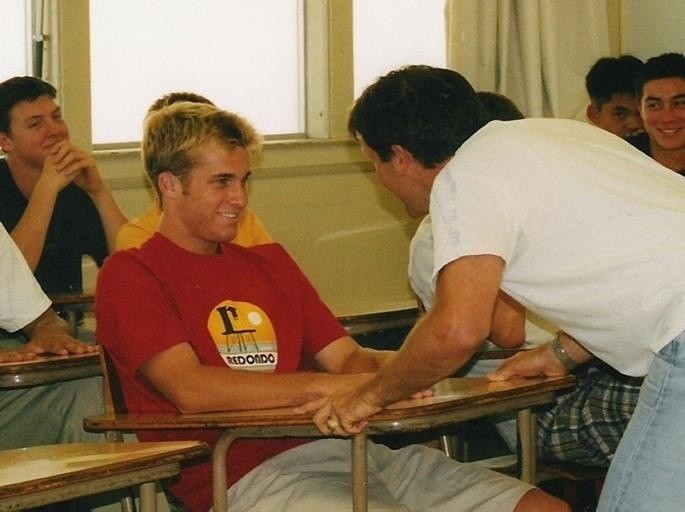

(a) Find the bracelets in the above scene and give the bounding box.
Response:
[550,328,581,374]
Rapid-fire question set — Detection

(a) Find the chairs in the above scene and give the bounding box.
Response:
[94,339,141,512]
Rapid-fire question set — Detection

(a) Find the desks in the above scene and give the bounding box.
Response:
[333,297,421,341]
[50,292,97,337]
[2,438,214,512]
[0,349,100,389]
[82,374,580,511]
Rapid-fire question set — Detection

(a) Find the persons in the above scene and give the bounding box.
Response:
[115,91,276,252]
[1,222,101,364]
[293,62,685,512]
[620,53,685,177]
[94,100,576,511]
[0,77,171,510]
[404,89,646,470]
[583,54,646,140]
